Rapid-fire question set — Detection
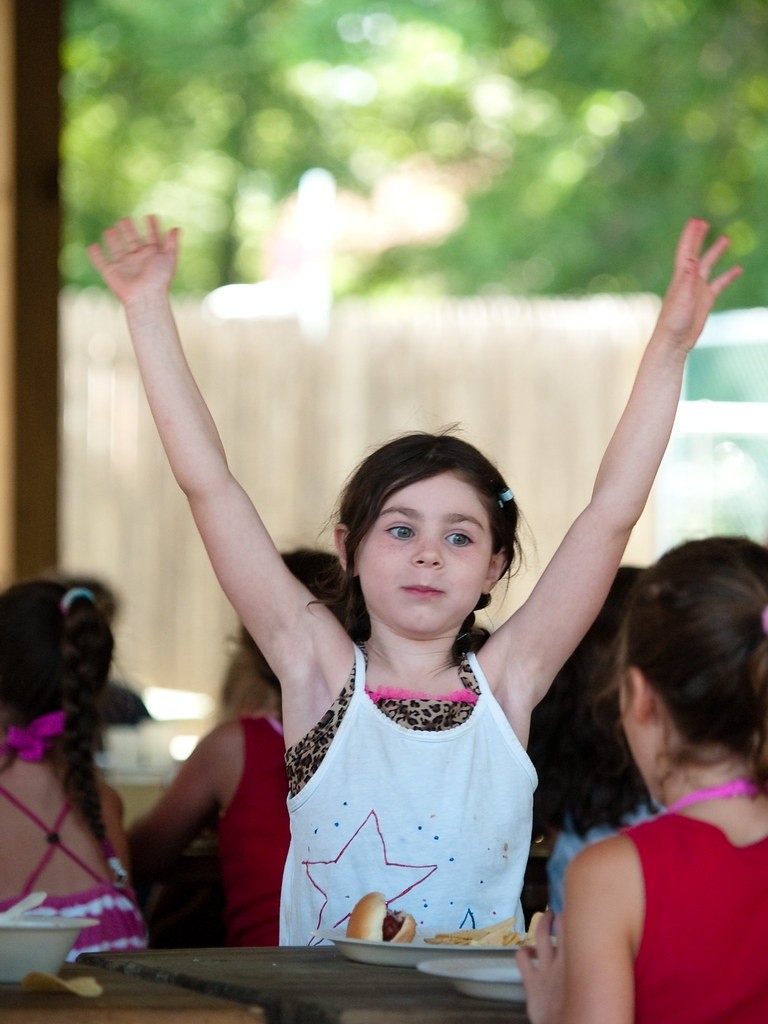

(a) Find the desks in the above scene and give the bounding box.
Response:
[0,944,534,1024]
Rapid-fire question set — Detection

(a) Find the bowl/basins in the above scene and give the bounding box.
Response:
[0,914,100,1024]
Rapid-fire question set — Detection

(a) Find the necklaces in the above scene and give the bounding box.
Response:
[667,779,757,813]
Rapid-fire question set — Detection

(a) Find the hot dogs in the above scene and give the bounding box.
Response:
[346,890,416,944]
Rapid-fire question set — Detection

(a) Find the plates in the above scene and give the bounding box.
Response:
[310,929,557,966]
[417,957,528,1001]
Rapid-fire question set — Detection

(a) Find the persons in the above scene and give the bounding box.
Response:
[89,213,743,946]
[0,577,153,962]
[129,550,349,943]
[516,536,768,1024]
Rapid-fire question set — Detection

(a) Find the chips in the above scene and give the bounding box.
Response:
[22,972,102,997]
[423,912,560,946]
